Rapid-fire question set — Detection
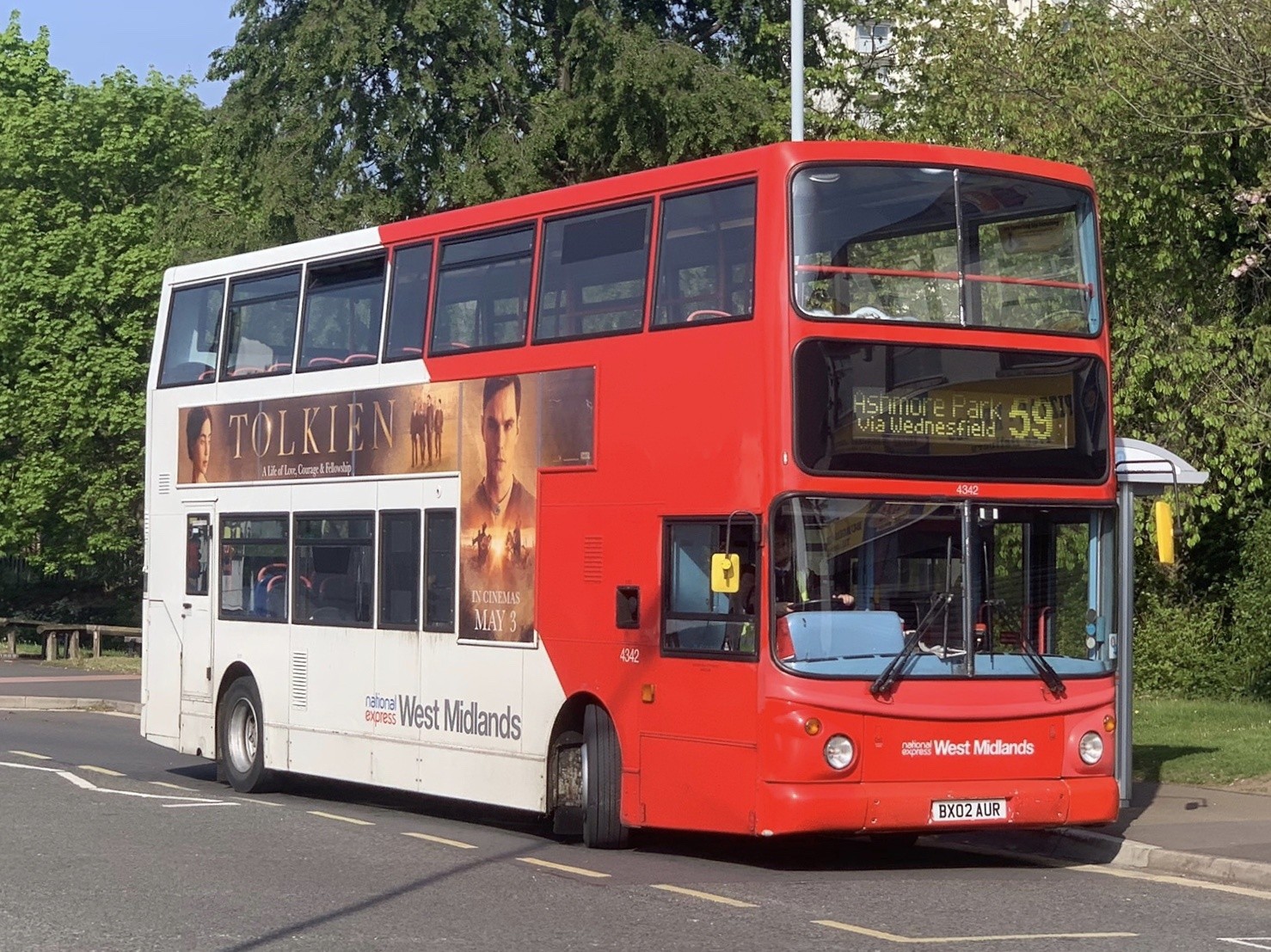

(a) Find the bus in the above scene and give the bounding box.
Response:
[139,139,1184,848]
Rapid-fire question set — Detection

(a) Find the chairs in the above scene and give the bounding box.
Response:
[176,309,747,389]
[237,560,449,632]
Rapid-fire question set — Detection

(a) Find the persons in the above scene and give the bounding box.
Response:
[412,395,446,469]
[184,407,213,483]
[462,374,537,643]
[741,526,855,619]
